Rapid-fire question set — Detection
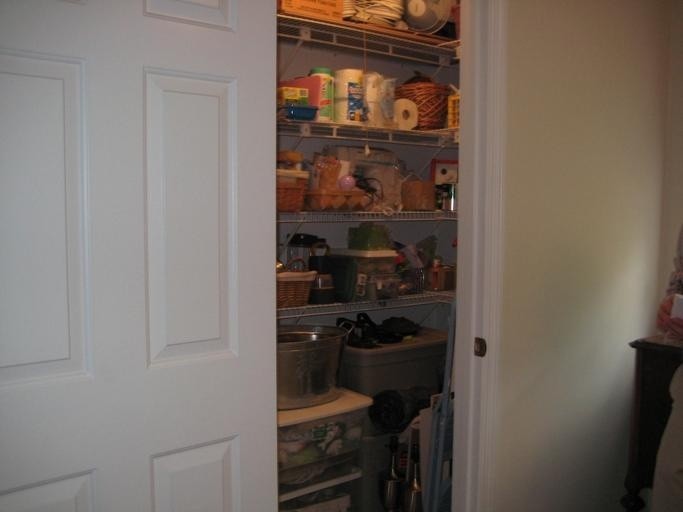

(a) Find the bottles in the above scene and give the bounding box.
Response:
[309,67,335,123]
[442,192,457,211]
[425,258,445,291]
[367,272,377,300]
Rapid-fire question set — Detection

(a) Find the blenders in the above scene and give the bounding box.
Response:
[286,232,335,303]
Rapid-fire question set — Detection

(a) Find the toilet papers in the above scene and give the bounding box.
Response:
[361,70,384,127]
[334,68,366,125]
[395,98,418,130]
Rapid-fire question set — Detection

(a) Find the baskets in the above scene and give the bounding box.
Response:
[393,80,451,132]
[276,165,310,212]
[276,255,319,308]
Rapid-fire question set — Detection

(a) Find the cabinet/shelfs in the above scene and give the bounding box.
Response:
[276,0,461,511]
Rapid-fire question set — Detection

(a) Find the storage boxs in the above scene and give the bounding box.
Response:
[278,392,372,473]
[328,248,398,299]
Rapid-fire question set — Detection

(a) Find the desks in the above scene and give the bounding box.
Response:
[618,334,683,512]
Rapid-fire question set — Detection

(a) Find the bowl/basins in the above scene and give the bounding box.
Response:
[287,105,318,121]
